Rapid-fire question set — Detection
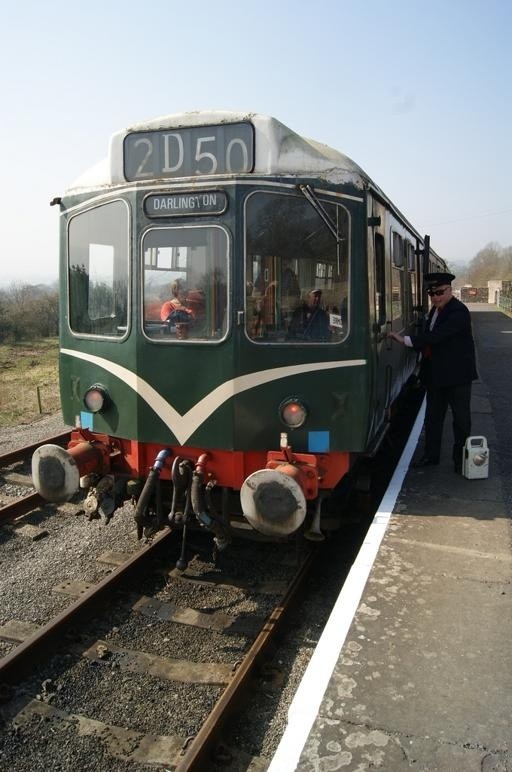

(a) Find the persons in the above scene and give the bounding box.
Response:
[157,276,205,323]
[282,286,332,343]
[386,269,478,475]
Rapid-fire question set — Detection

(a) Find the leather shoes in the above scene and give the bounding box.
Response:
[408,457,439,468]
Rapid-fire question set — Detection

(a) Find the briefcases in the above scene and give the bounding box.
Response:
[461,435,490,480]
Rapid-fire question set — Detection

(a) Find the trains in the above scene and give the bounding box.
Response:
[31,111,451,571]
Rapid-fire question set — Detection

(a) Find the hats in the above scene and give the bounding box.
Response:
[422,270,456,287]
[305,287,321,297]
